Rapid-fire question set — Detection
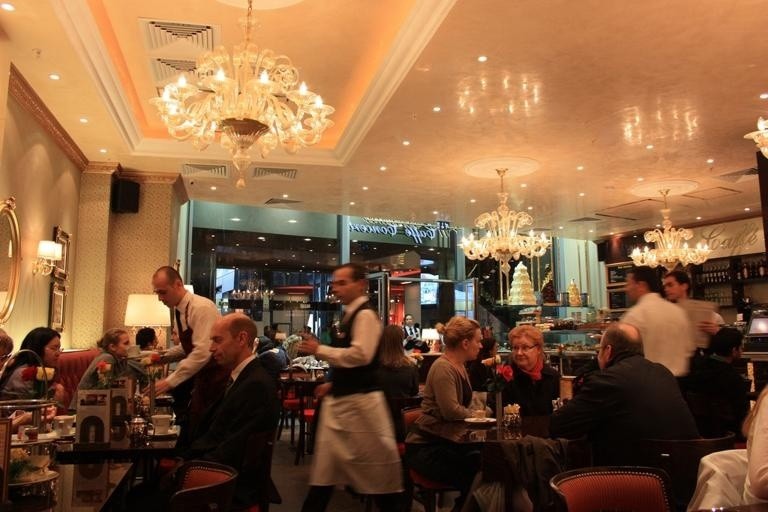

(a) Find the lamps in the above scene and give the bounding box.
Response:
[460,157,552,279]
[148,0,336,190]
[124,294,171,345]
[32,240,62,277]
[628,180,713,275]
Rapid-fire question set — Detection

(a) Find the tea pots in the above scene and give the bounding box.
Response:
[124,414,155,445]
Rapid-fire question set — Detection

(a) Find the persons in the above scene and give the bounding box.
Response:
[295,264,768,512]
[1,261,295,511]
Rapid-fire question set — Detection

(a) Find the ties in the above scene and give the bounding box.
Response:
[409,328,413,335]
[222,377,234,397]
[174,308,183,336]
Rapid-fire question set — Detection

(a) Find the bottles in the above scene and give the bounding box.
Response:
[737,259,765,280]
[704,289,732,305]
[702,264,731,282]
[589,306,596,322]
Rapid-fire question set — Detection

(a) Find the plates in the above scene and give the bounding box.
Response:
[6,471,60,486]
[146,430,179,439]
[51,429,76,436]
[464,416,498,425]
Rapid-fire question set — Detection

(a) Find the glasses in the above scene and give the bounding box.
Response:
[0,354,12,363]
[595,346,607,353]
[511,343,540,351]
[45,346,64,354]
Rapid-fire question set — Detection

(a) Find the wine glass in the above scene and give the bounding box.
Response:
[29,442,52,477]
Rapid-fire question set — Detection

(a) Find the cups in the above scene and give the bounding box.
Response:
[586,313,593,322]
[52,414,76,435]
[149,414,172,433]
[24,427,39,443]
[472,406,485,419]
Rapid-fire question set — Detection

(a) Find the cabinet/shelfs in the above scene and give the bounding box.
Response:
[538,304,610,373]
[605,251,767,334]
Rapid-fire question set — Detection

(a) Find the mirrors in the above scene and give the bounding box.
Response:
[0,193,22,326]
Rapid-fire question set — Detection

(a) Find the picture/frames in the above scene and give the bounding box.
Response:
[48,282,67,333]
[53,224,70,281]
